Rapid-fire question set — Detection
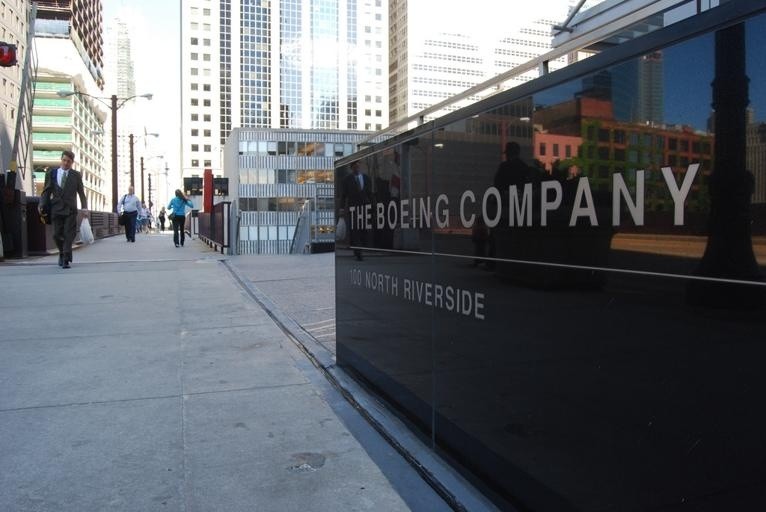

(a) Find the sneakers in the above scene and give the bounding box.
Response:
[125,237,136,243]
[174,241,184,247]
[57,254,72,269]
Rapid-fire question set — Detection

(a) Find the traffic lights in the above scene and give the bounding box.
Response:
[0,41,18,67]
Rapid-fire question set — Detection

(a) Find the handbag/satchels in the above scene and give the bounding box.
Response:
[38,193,52,225]
[168,213,176,220]
[119,215,125,225]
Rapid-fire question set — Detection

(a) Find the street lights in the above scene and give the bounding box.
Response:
[417,141,446,194]
[471,114,529,173]
[57,89,170,225]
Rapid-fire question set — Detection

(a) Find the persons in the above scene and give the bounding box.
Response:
[39,150,89,268]
[167,189,193,247]
[136,203,166,234]
[340,160,372,261]
[470,141,546,272]
[116,186,143,242]
